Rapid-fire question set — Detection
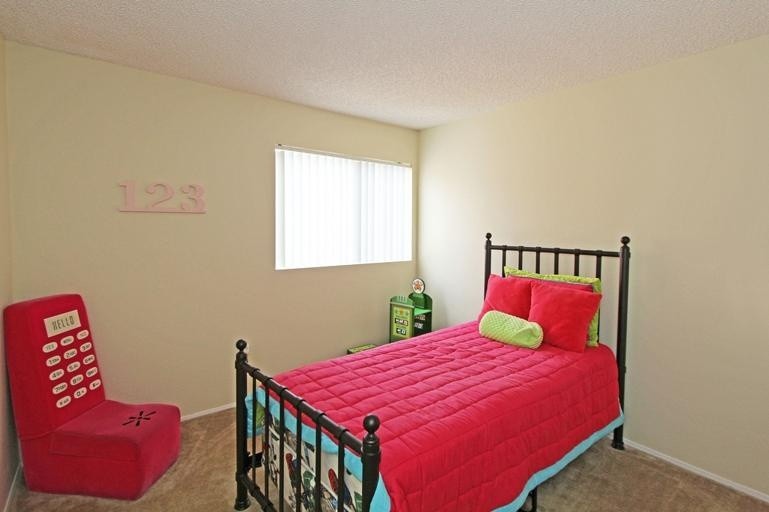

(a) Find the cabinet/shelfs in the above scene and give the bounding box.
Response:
[388,278,433,343]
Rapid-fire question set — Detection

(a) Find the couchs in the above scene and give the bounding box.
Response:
[2,293,182,502]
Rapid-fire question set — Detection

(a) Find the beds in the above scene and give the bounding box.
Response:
[233,232,633,512]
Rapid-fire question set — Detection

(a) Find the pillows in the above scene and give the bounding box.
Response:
[477,274,532,324]
[478,310,544,351]
[507,274,594,293]
[504,265,602,348]
[531,281,603,353]
